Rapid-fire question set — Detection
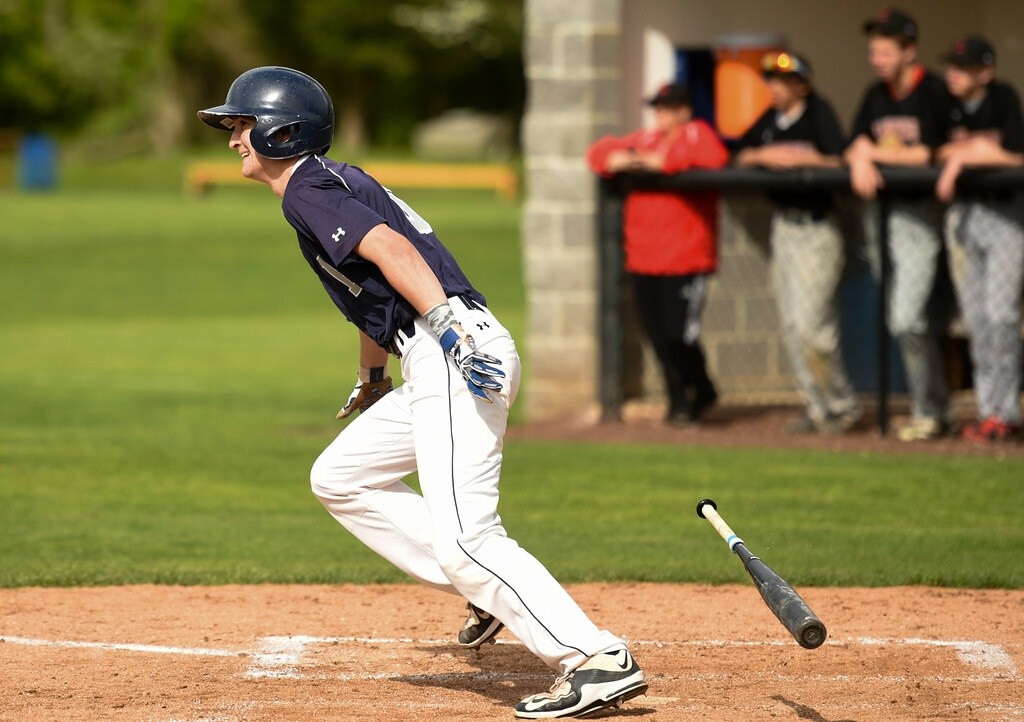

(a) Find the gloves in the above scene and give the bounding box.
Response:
[336,363,394,421]
[440,322,506,404]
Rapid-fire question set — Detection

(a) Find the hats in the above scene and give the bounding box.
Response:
[861,11,916,44]
[940,34,995,70]
[755,51,809,81]
[649,85,694,106]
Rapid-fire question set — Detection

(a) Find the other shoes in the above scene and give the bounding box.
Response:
[782,403,863,437]
[897,417,942,442]
[663,384,720,428]
[964,417,1013,447]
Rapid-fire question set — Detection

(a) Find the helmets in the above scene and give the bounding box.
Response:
[198,66,334,160]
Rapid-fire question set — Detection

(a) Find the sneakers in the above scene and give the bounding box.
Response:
[459,601,506,648]
[513,649,649,719]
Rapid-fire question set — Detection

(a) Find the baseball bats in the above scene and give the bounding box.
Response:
[695,495,829,651]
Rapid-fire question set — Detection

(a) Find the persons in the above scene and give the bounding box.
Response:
[197,68,647,717]
[728,46,865,436]
[936,31,1024,445]
[587,80,731,428]
[840,7,958,443]
